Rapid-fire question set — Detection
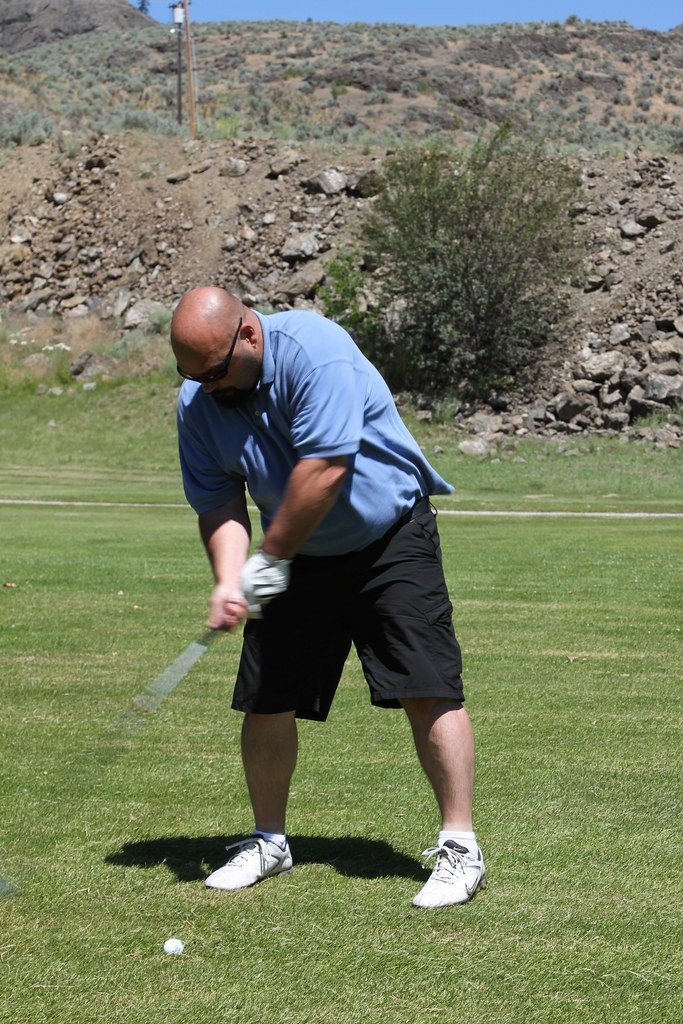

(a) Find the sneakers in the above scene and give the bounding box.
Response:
[204,834,294,890]
[411,839,485,909]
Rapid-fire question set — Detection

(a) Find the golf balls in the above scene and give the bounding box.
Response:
[163,938,184,955]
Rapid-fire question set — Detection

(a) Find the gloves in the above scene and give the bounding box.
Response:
[238,551,292,611]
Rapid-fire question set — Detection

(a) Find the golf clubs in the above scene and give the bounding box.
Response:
[1,626,228,897]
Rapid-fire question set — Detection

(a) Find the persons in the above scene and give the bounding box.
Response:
[170,287,487,909]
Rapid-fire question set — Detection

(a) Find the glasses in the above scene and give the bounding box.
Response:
[176,317,243,383]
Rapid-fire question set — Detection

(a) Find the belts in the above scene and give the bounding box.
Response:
[385,496,431,534]
